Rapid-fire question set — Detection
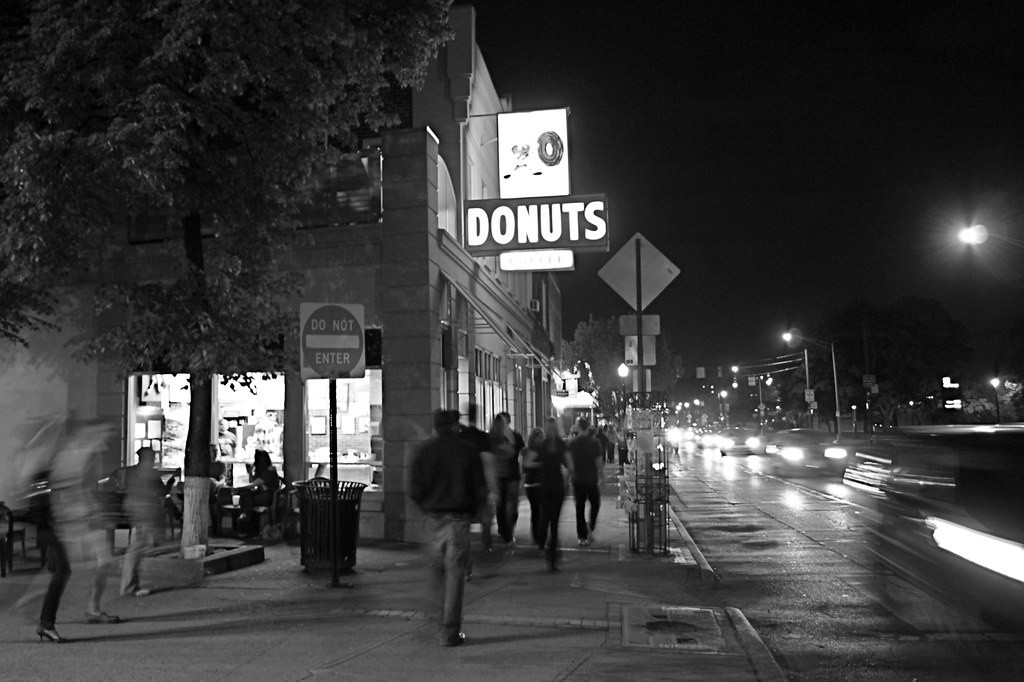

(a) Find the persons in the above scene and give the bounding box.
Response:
[462,401,617,579]
[17,411,73,642]
[118,448,182,597]
[232,449,280,535]
[51,422,121,622]
[176,444,226,536]
[409,410,477,646]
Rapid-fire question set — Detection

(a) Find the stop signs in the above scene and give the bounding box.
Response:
[301,303,365,379]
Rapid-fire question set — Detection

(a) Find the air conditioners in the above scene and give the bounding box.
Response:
[531,300,540,312]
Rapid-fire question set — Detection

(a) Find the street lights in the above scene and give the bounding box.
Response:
[908,398,915,427]
[851,405,858,438]
[782,332,841,437]
[618,359,631,466]
[990,377,1001,425]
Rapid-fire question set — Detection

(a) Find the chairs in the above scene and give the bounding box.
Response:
[0,467,291,580]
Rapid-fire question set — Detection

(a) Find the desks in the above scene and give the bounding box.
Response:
[223,504,243,536]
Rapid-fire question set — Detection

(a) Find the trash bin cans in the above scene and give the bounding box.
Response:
[292,479,369,572]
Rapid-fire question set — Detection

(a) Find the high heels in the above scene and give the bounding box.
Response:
[36,625,70,643]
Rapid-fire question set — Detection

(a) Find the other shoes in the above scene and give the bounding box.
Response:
[504,536,518,548]
[550,565,563,573]
[441,632,467,645]
[131,587,152,596]
[85,611,120,623]
[578,537,590,546]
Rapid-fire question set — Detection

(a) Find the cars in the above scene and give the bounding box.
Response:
[658,424,1024,641]
